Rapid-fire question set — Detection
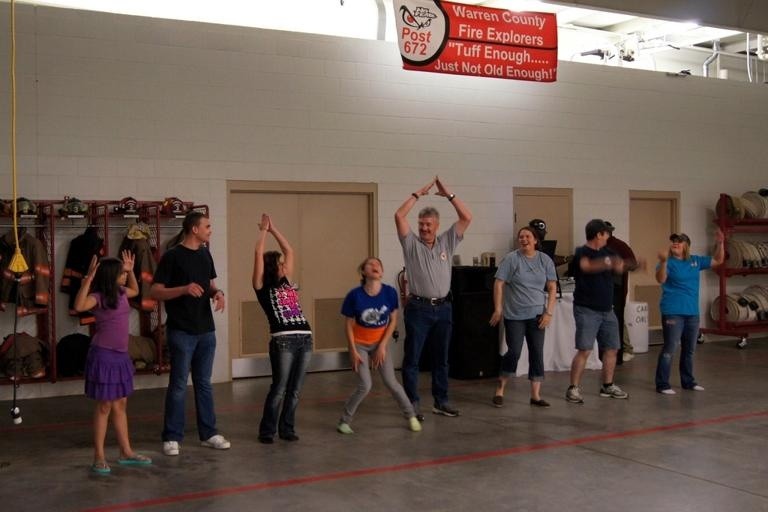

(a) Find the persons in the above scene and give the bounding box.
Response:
[395,176,473,421]
[489,227,558,408]
[655,226,725,394]
[151,211,231,456]
[599,221,636,365]
[565,219,628,404]
[252,213,313,444]
[336,257,423,434]
[74,249,153,473]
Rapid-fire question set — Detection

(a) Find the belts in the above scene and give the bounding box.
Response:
[410,293,452,306]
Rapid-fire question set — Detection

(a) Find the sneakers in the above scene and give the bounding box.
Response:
[163,441,180,456]
[416,415,425,421]
[493,391,504,408]
[530,398,551,408]
[432,402,462,417]
[201,434,231,449]
[599,383,629,399]
[565,386,585,405]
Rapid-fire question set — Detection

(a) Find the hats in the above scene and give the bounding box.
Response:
[670,233,691,247]
[586,219,615,236]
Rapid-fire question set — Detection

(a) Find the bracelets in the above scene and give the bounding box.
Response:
[214,289,224,296]
[82,275,93,282]
[448,193,456,201]
[412,193,419,200]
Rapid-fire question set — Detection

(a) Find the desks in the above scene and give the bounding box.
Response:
[499,293,603,376]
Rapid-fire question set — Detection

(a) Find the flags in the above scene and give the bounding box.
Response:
[393,0,557,82]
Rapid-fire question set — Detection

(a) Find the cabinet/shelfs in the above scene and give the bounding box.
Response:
[693,190,768,345]
[0,196,211,386]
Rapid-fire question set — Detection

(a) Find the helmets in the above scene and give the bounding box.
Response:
[17,198,39,215]
[58,198,89,214]
[114,197,138,212]
[160,198,189,215]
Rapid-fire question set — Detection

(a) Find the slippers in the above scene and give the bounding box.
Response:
[91,463,113,473]
[117,453,153,465]
